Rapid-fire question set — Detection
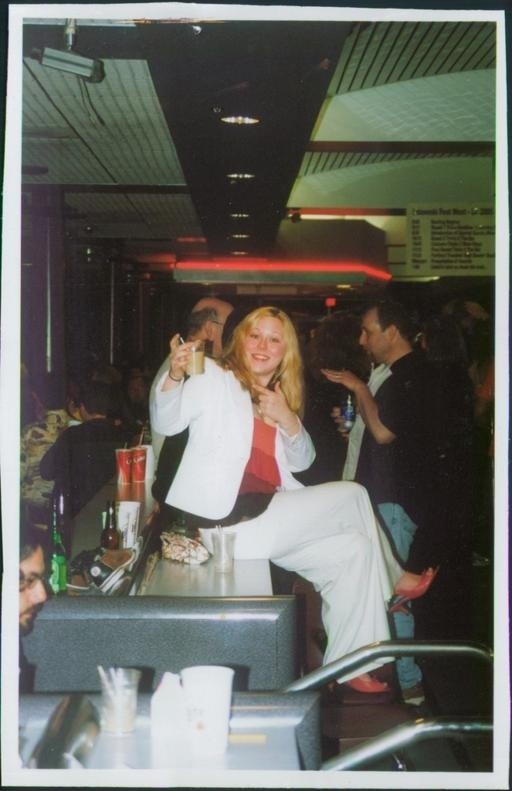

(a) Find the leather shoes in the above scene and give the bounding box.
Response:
[90,548,136,596]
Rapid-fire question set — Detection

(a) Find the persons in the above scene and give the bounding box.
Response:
[152,310,301,597]
[300,284,493,652]
[20,343,157,521]
[19,568,33,634]
[149,303,442,695]
[21,531,46,603]
[147,297,235,496]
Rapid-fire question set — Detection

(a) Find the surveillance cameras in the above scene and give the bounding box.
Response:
[28,43,106,90]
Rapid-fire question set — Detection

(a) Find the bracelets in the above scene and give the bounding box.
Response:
[167,366,182,380]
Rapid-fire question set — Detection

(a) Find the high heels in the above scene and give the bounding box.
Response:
[387,566,439,615]
[345,674,391,693]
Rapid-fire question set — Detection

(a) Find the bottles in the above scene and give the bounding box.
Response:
[339,386,356,429]
[41,495,69,596]
[100,498,120,550]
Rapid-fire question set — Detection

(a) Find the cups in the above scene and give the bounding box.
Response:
[210,530,236,572]
[179,663,235,751]
[184,341,208,376]
[100,667,139,733]
[102,500,139,547]
[115,448,134,484]
[131,445,150,482]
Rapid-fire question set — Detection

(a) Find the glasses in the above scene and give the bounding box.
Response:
[20,570,53,588]
